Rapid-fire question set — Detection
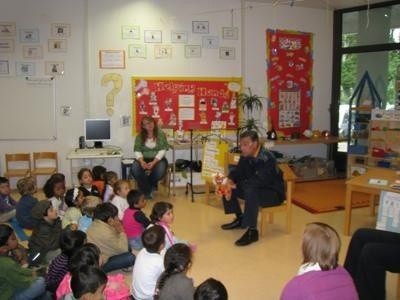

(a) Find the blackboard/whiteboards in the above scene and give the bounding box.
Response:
[0,75,57,141]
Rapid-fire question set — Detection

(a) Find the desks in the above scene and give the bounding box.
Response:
[263,136,349,161]
[345,167,400,237]
[67,149,122,187]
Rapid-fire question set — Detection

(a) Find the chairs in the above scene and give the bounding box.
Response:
[5,153,32,185]
[259,181,292,236]
[32,151,59,189]
[127,160,171,198]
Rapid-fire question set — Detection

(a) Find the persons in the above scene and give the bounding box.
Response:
[0,165,195,300]
[217,131,284,246]
[344,228,400,300]
[279,222,360,300]
[194,278,228,300]
[131,117,169,200]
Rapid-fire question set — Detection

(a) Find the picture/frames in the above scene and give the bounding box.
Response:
[0,19,72,76]
[121,21,238,61]
[99,49,125,68]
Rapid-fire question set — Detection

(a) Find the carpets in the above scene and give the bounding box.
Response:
[283,177,379,214]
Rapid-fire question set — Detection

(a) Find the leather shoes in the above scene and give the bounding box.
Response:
[221,217,246,229]
[235,228,258,245]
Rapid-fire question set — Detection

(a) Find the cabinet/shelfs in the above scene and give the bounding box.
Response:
[347,106,372,164]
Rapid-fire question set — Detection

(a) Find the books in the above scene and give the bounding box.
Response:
[346,105,400,178]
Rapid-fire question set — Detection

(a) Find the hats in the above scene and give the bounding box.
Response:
[32,199,51,219]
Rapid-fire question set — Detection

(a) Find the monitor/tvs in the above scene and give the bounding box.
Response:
[84,118,112,148]
